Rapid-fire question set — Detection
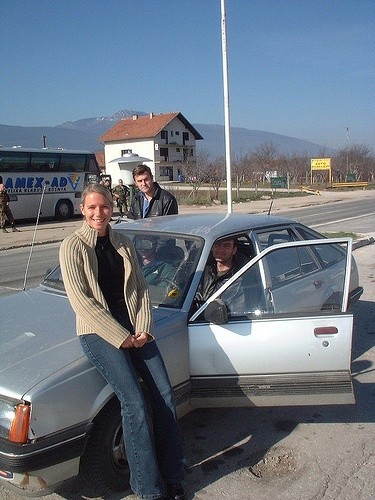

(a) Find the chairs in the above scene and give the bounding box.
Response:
[265,234,292,276]
[157,247,185,262]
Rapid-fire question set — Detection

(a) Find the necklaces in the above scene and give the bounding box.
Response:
[96,236,109,250]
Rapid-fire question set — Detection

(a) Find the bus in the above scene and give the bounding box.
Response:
[0,146,102,221]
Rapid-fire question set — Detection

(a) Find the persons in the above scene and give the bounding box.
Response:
[127,165,179,267]
[134,234,261,316]
[0,183,22,233]
[99,179,141,221]
[59,184,188,500]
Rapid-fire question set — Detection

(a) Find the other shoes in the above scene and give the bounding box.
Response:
[12,228,18,232]
[3,229,8,233]
[121,215,123,218]
[167,483,185,500]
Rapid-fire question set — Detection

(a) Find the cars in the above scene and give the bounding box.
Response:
[0,211,364,499]
